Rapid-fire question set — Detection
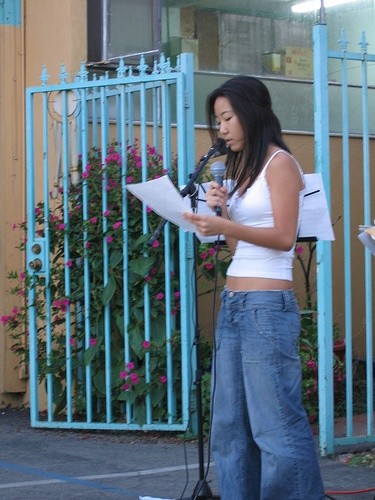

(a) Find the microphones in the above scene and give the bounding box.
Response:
[211,162,226,217]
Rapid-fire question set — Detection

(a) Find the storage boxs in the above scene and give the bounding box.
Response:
[170,35,199,72]
[272,46,314,80]
[261,51,282,76]
